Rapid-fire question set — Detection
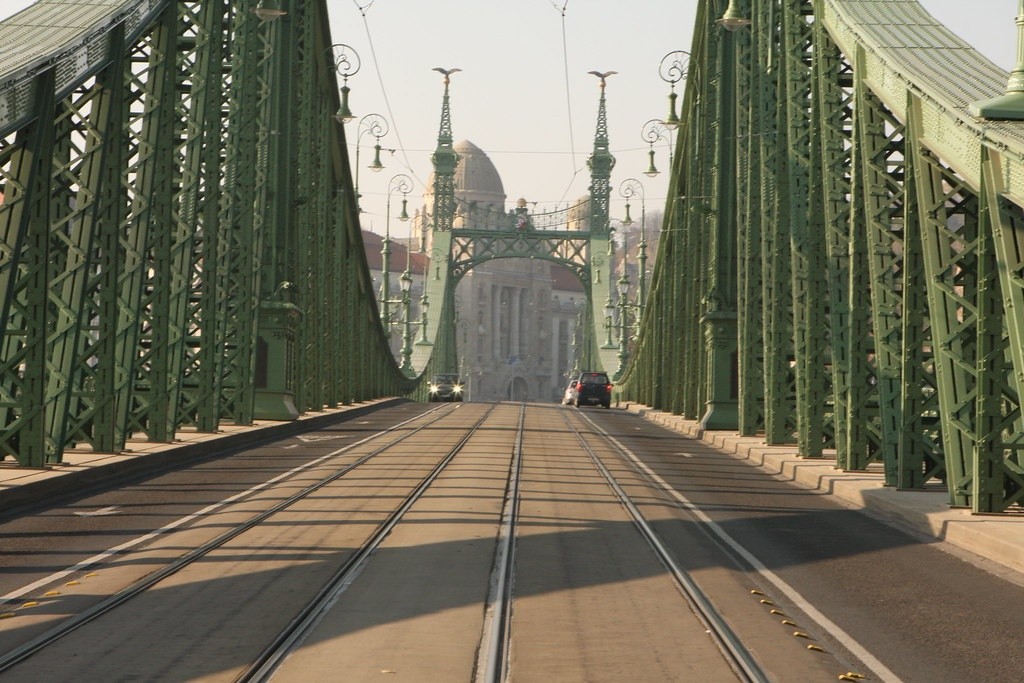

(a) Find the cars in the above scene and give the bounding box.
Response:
[562,380,600,406]
[573,372,614,409]
[427,373,465,402]
